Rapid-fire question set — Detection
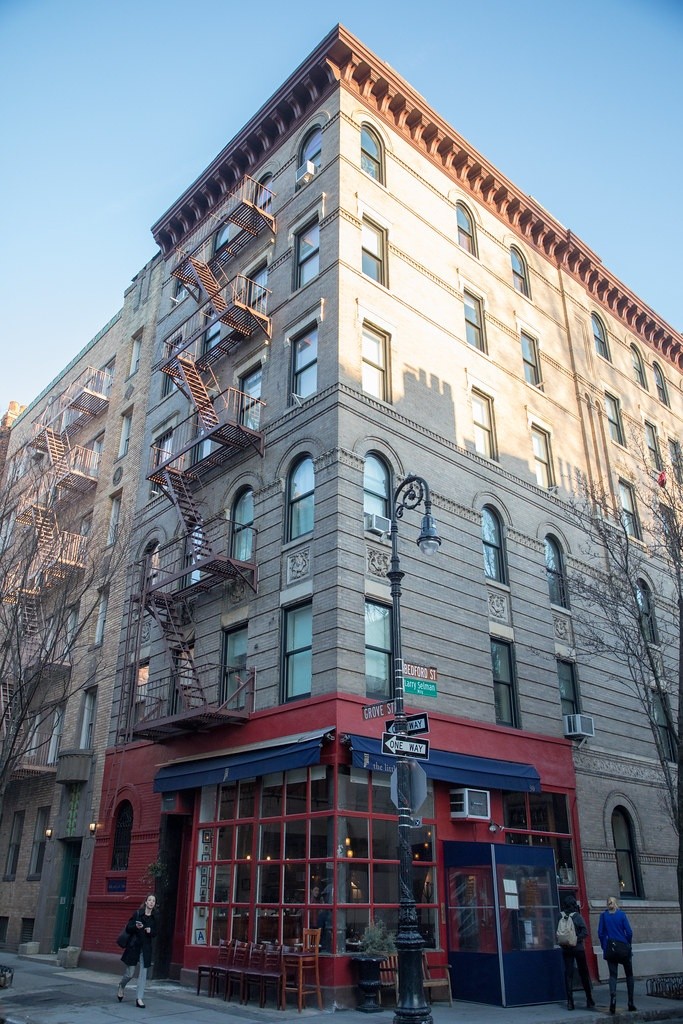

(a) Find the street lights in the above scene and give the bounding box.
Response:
[385,472,442,1024]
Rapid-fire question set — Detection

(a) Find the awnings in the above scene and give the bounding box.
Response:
[349,736,541,792]
[153,725,336,794]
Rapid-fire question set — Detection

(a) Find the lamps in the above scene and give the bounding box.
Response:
[489,820,505,833]
[45,830,52,838]
[90,823,96,834]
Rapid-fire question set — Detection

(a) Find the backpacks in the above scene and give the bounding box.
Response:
[557,911,578,946]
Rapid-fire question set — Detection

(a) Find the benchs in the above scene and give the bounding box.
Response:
[377,950,453,1007]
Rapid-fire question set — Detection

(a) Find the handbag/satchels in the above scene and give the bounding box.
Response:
[606,939,632,964]
[116,910,140,949]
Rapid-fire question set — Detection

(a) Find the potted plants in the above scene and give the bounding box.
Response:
[350,921,397,1013]
[140,862,168,888]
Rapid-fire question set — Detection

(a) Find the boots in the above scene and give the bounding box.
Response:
[628,994,637,1011]
[610,993,616,1013]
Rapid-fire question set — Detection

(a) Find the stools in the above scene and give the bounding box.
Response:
[281,928,322,1011]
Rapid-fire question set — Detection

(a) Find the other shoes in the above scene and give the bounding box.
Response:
[117,995,124,1002]
[567,1002,575,1010]
[587,999,595,1009]
[136,999,146,1008]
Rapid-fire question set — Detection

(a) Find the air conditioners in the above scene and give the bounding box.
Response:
[295,160,317,187]
[563,714,595,748]
[364,514,391,536]
[449,788,491,819]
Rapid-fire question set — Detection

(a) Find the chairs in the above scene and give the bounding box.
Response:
[197,928,297,1010]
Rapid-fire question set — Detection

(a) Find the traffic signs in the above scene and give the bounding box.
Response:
[385,712,430,737]
[380,732,430,761]
[361,699,395,721]
[403,661,437,681]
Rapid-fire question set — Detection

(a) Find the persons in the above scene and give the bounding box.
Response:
[556,896,595,1010]
[310,886,320,929]
[118,895,161,1007]
[598,897,637,1014]
